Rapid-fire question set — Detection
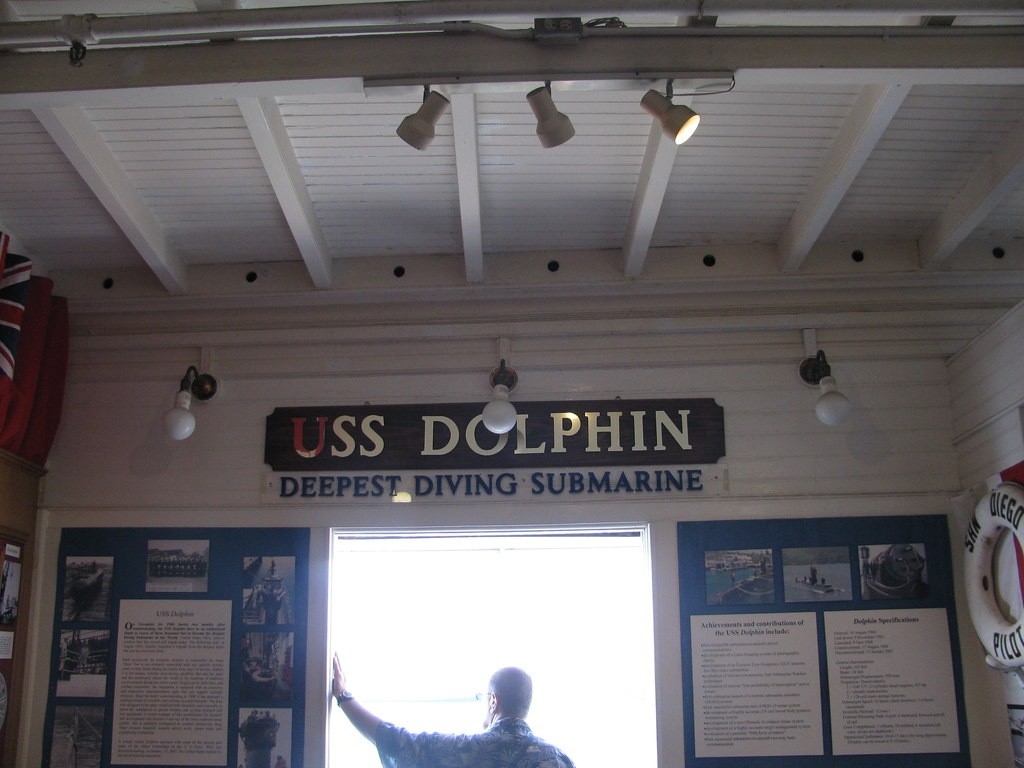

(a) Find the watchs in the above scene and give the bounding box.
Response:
[336,690,355,707]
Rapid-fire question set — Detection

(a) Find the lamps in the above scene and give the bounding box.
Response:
[395,85,451,150]
[639,85,701,145]
[526,81,575,148]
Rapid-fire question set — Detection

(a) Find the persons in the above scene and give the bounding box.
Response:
[730,569,737,587]
[332,652,576,768]
[810,566,818,586]
[150,555,207,577]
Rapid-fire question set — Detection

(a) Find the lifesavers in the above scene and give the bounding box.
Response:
[961,478,1024,668]
